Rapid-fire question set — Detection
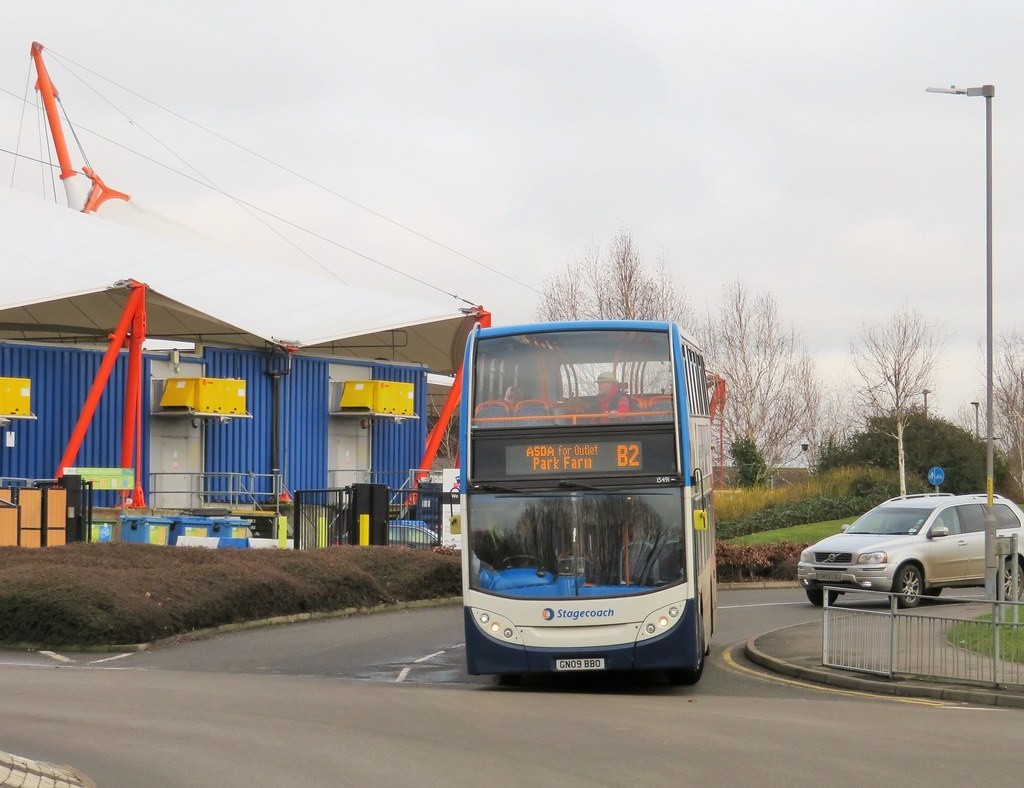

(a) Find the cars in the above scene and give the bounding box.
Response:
[332,521,441,549]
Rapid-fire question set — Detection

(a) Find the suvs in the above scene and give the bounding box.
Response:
[799,493,1024,609]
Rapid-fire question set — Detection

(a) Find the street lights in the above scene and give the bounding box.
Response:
[970,402,980,443]
[923,390,930,412]
[928,85,996,604]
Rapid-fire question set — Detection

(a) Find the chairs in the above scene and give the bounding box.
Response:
[472,394,674,428]
[619,541,684,586]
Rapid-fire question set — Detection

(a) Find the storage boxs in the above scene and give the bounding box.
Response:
[119,515,175,547]
[163,515,214,546]
[209,519,252,538]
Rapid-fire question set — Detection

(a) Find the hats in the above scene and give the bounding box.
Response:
[594,372,618,383]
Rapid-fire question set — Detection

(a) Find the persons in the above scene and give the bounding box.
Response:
[596,372,629,414]
[492,534,535,569]
[504,386,524,403]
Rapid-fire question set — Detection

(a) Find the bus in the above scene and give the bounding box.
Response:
[459,319,720,688]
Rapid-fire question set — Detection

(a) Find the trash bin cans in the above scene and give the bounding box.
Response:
[85,519,117,543]
[211,518,251,548]
[161,516,213,546]
[120,515,173,545]
